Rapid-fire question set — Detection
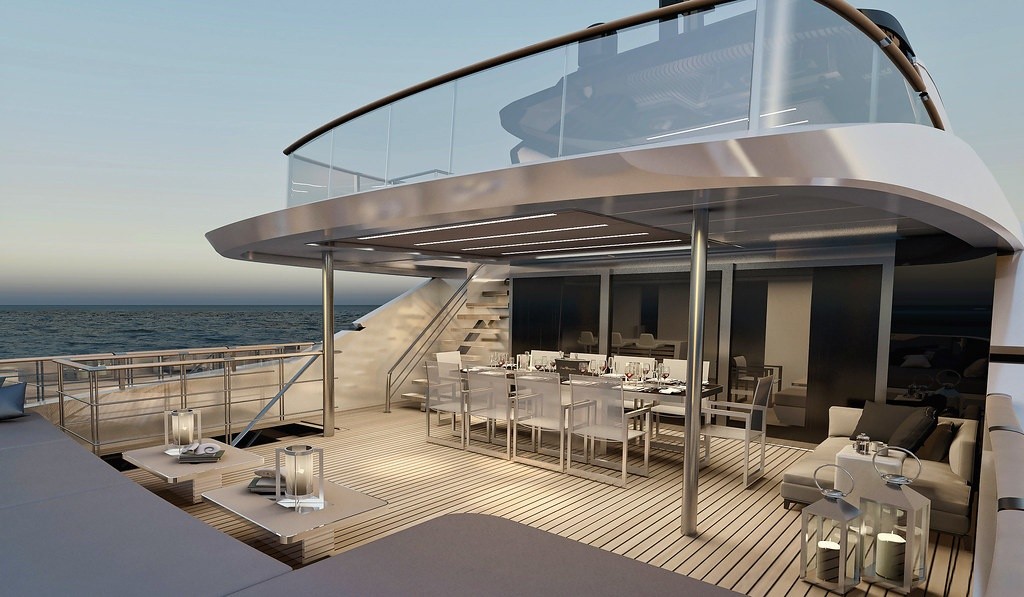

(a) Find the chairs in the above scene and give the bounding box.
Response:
[733,356,782,405]
[425,349,776,490]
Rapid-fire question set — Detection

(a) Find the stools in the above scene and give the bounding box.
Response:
[578,331,599,353]
[612,332,633,356]
[636,333,665,357]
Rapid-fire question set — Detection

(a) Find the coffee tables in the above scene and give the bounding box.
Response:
[123,437,263,503]
[202,470,389,564]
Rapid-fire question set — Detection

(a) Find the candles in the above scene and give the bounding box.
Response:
[875,530,906,579]
[817,537,840,583]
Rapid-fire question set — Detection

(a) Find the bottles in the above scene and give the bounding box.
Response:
[624,360,670,389]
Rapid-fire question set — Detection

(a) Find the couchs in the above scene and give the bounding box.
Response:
[0,412,751,596]
[774,383,807,426]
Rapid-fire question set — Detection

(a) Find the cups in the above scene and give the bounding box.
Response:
[557,352,564,360]
[870,441,878,451]
[877,442,888,456]
[570,353,578,358]
[853,432,870,454]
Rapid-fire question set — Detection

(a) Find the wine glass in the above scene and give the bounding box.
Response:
[590,356,619,377]
[542,355,547,372]
[489,352,515,372]
[549,356,556,371]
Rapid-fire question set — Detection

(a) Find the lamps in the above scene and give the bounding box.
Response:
[164,409,201,454]
[275,444,324,511]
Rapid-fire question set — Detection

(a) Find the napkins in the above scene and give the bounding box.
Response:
[462,369,479,371]
[612,385,644,391]
[659,387,682,394]
[479,371,504,376]
[517,376,546,380]
[562,380,598,385]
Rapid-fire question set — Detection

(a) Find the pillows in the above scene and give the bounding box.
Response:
[850,400,963,462]
[0,377,31,421]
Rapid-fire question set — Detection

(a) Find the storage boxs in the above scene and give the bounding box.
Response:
[555,359,590,377]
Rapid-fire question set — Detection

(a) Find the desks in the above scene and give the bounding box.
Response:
[595,338,687,363]
[450,364,723,471]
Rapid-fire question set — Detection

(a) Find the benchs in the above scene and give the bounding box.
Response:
[780,406,979,538]
